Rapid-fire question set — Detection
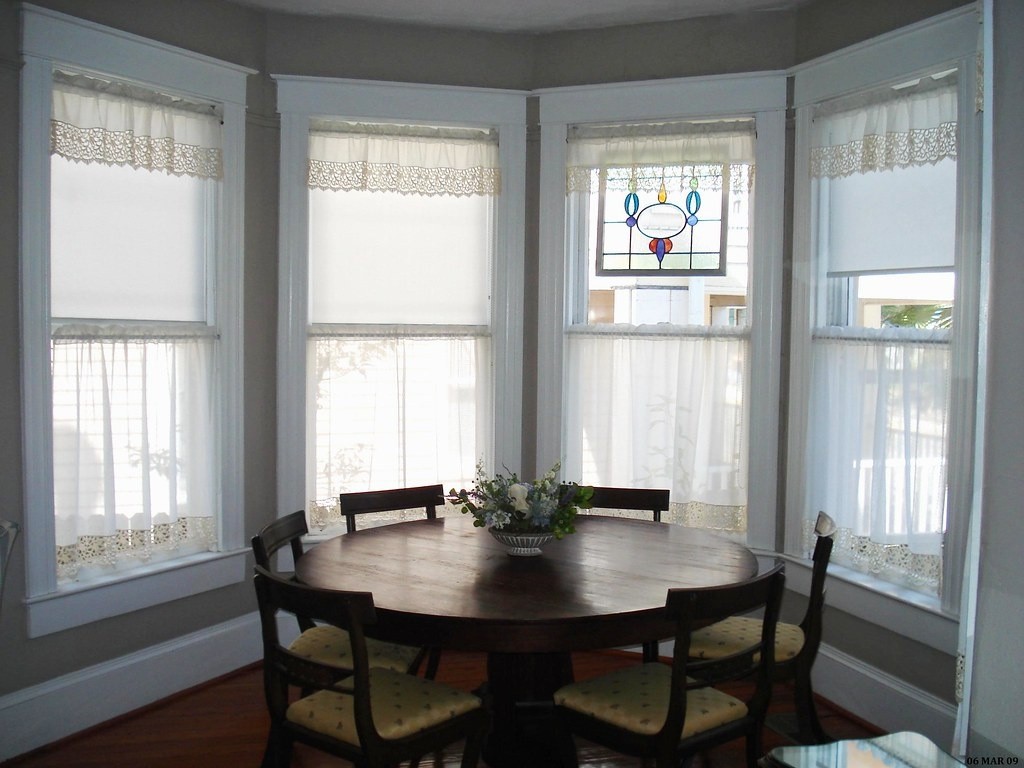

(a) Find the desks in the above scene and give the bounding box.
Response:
[294,515,758,768]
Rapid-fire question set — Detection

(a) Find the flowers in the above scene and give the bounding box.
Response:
[437,451,595,540]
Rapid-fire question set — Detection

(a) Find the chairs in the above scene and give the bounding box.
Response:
[250,484,838,768]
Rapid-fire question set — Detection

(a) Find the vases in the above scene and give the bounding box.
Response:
[488,527,557,556]
